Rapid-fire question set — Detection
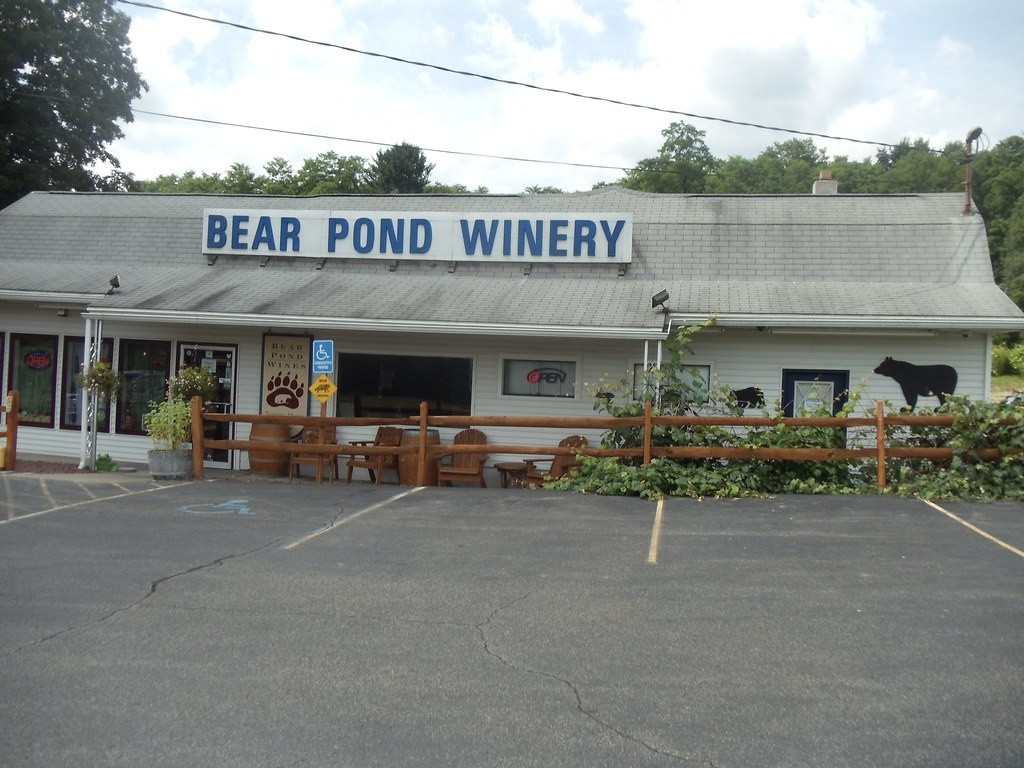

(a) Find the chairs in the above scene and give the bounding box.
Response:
[434,428,490,489]
[523,435,588,487]
[346,427,403,486]
[289,425,338,483]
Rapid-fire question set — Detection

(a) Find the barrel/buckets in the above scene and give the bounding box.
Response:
[248,422,291,478]
[398,429,441,486]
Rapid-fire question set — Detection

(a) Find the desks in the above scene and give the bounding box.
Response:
[495,462,537,488]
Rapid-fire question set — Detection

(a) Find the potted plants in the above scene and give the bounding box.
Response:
[145,398,193,480]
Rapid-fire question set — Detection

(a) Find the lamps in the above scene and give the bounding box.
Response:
[107,275,121,295]
[652,289,670,313]
